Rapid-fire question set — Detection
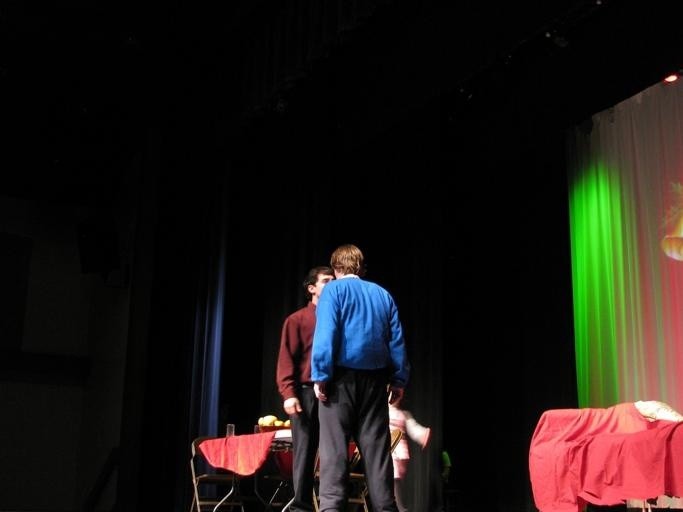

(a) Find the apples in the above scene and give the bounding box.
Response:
[258,415,290,427]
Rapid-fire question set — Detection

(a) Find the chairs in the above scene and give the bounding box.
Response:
[311,430,402,512]
[189,436,245,512]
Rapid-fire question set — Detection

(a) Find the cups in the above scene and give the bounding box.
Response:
[226,424,234,438]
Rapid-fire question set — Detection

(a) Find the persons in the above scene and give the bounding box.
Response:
[274,265,335,511]
[388,384,433,512]
[309,243,413,512]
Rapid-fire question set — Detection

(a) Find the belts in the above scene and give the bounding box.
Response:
[302,384,313,389]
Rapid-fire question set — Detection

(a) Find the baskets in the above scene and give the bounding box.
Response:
[260,426,291,432]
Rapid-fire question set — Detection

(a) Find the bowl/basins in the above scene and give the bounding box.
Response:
[258,425,291,433]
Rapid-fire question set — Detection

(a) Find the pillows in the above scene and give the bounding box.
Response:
[635,400,683,422]
